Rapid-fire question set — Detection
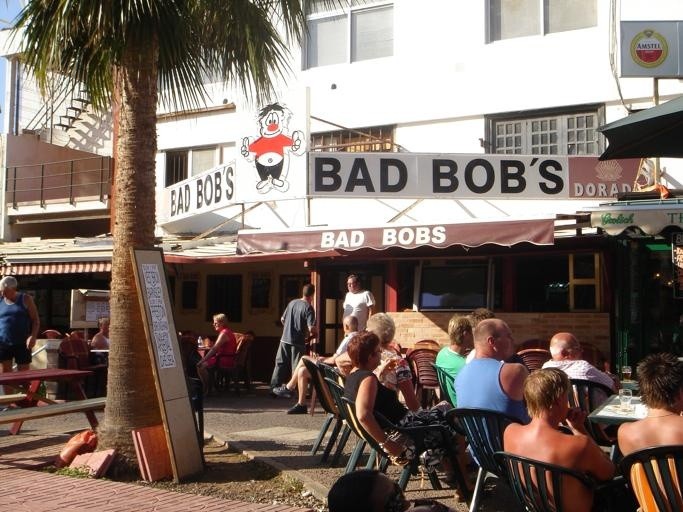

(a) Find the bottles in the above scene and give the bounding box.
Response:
[197,336,202,347]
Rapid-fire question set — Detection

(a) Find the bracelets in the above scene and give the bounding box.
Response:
[377,436,391,450]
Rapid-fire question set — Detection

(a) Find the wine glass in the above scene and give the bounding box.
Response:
[621,365,631,382]
[618,388,632,409]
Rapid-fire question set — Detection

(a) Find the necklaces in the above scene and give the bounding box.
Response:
[645,414,676,418]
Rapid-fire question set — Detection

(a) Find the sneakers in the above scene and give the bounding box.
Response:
[296,393,312,399]
[269,384,280,400]
[287,403,307,414]
[272,384,294,398]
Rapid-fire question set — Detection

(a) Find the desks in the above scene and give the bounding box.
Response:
[588,377,649,446]
[0,367,99,436]
[90,349,109,353]
[196,340,214,358]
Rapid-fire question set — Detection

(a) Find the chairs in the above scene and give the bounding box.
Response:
[205,330,256,397]
[40,329,108,399]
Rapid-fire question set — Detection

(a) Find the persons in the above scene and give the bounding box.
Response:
[470,308,495,327]
[617,353,683,512]
[196,313,236,397]
[328,469,460,512]
[540,333,624,464]
[0,276,41,413]
[435,315,477,409]
[368,313,421,410]
[346,330,477,503]
[455,318,530,472]
[343,275,376,332]
[502,369,639,512]
[268,282,316,399]
[91,317,111,365]
[272,315,362,414]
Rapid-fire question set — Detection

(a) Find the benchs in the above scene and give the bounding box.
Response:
[0,397,106,424]
[0,393,27,405]
